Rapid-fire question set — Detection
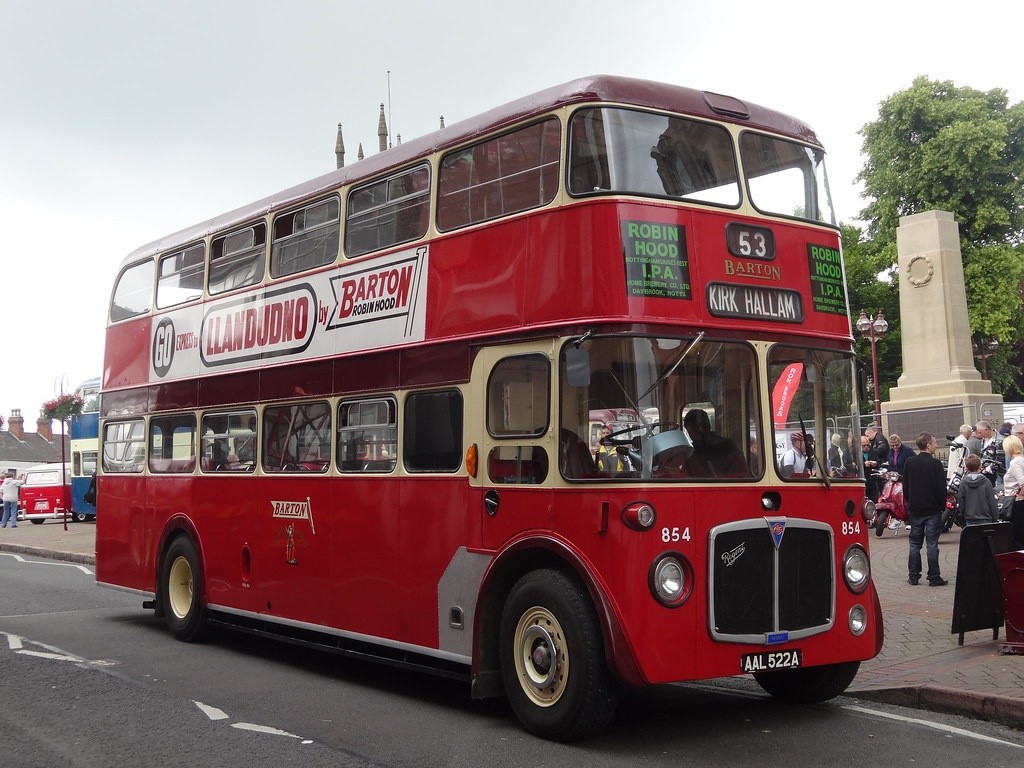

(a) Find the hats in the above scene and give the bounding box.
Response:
[1012,423,1024,432]
[999,428,1011,436]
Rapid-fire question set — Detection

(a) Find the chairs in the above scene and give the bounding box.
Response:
[216,460,392,473]
[532,428,597,484]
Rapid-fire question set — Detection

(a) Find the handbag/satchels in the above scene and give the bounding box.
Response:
[83,492,95,505]
[995,496,1015,521]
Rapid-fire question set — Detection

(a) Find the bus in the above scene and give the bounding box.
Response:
[94,74,885,735]
[71,377,102,521]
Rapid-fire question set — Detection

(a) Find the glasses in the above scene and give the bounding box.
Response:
[891,444,898,446]
[861,445,869,447]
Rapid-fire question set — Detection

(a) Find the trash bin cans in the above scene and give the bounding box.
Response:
[994,550,1024,654]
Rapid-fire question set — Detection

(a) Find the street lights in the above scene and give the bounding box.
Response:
[857,308,889,433]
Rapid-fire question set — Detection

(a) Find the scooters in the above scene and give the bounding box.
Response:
[871,462,909,538]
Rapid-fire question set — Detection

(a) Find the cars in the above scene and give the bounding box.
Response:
[17,462,72,524]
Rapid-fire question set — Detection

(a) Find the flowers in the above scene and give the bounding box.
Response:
[43,393,85,421]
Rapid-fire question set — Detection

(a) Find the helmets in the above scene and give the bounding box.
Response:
[790,432,804,441]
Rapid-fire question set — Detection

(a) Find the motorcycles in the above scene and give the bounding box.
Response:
[942,435,1002,533]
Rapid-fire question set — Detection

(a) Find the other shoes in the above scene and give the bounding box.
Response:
[907,579,918,585]
[888,520,902,530]
[929,579,948,586]
[905,525,911,531]
[11,525,19,529]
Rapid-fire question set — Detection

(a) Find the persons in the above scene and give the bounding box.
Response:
[997,427,1010,485]
[680,408,747,479]
[842,436,880,504]
[958,454,999,529]
[903,431,948,586]
[865,428,890,472]
[1003,435,1024,522]
[0,472,26,527]
[1011,423,1024,448]
[749,436,759,479]
[828,433,843,477]
[90,470,97,507]
[595,426,630,472]
[780,432,814,477]
[947,424,972,478]
[964,426,981,458]
[976,421,1005,488]
[887,434,918,476]
[1003,422,1012,430]
